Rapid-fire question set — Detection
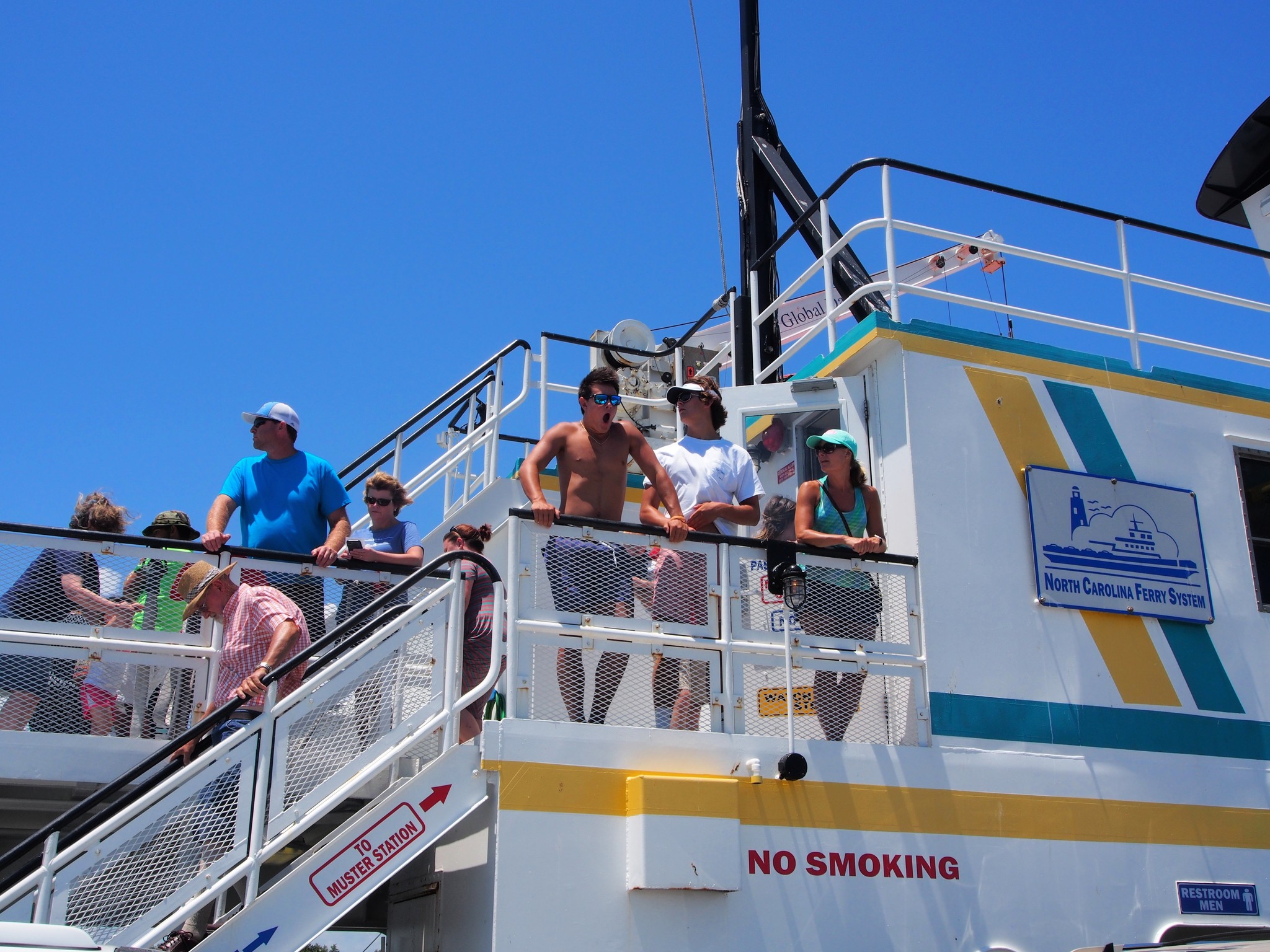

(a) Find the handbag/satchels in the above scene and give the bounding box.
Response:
[871,585,883,613]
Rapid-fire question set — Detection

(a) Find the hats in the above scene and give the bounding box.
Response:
[806,429,857,461]
[176,560,238,622]
[666,383,719,405]
[242,402,300,433]
[142,510,200,541]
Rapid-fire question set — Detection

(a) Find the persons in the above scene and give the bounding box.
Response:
[0,402,509,952]
[519,365,887,741]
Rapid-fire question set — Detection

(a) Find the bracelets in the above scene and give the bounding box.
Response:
[670,516,687,524]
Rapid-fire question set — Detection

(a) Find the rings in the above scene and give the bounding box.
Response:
[330,555,334,559]
[360,555,362,559]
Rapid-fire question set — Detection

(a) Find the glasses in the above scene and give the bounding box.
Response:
[449,525,464,543]
[671,391,702,404]
[588,394,621,406]
[198,590,209,613]
[254,417,274,428]
[366,495,394,506]
[814,441,848,458]
[152,528,162,535]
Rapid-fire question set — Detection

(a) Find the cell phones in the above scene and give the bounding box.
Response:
[346,540,363,554]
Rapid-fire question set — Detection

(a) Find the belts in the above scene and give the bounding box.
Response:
[217,711,258,721]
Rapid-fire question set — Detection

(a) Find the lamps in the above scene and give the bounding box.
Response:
[772,562,807,609]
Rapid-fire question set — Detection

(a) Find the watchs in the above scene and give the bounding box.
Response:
[871,534,883,547]
[253,662,272,674]
